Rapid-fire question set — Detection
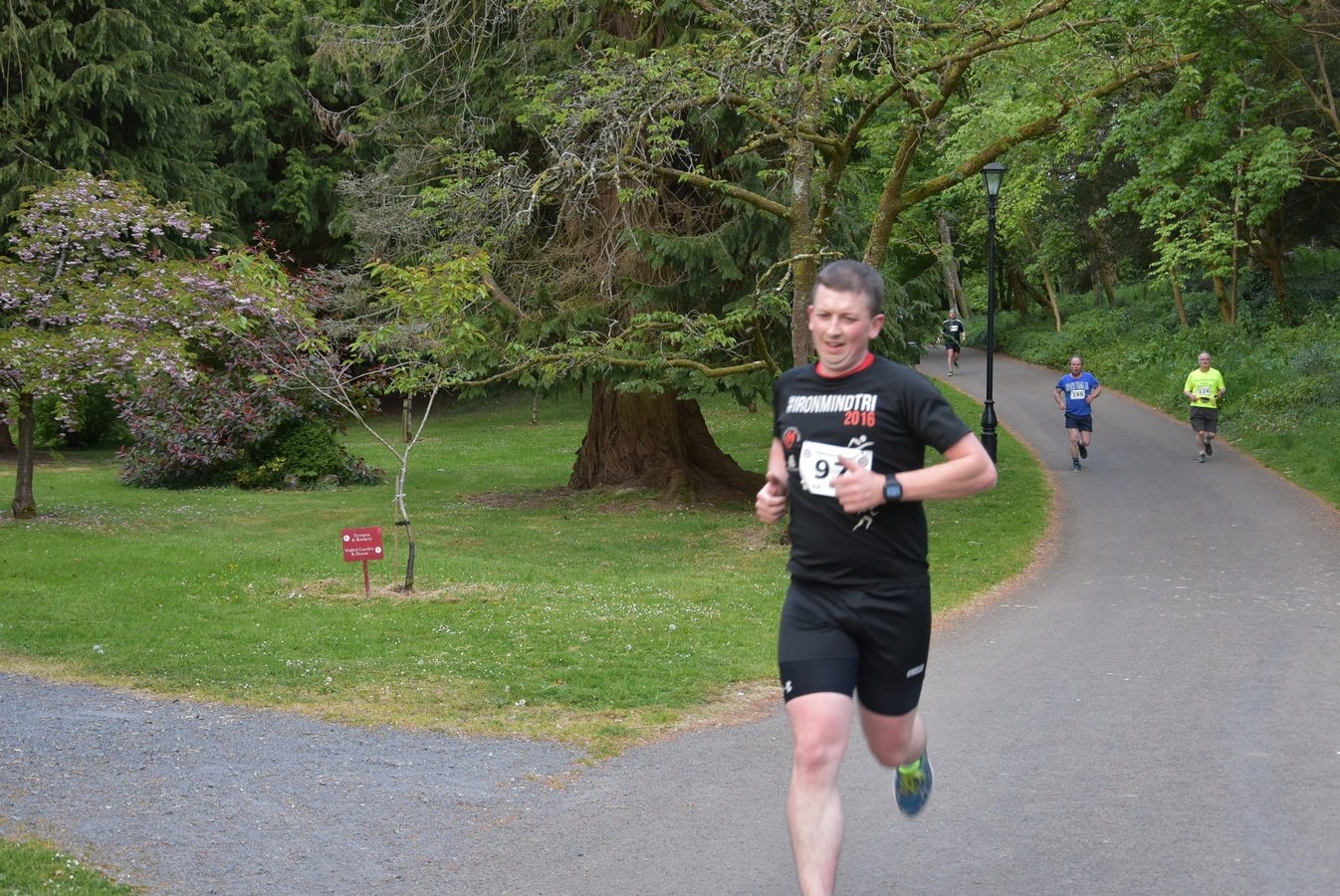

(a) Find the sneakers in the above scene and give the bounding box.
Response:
[1197,454,1206,462]
[1205,443,1212,455]
[894,750,932,818]
[1078,441,1087,457]
[1072,463,1081,472]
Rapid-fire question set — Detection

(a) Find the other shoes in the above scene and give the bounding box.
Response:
[954,362,958,367]
[948,372,952,376]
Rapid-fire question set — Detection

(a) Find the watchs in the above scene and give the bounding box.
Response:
[883,472,903,506]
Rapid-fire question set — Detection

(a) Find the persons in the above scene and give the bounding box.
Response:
[754,260,996,896]
[1053,356,1102,472]
[1183,353,1226,463]
[936,309,965,377]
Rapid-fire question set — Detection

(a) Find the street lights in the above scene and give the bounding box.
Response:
[978,163,1009,465]
